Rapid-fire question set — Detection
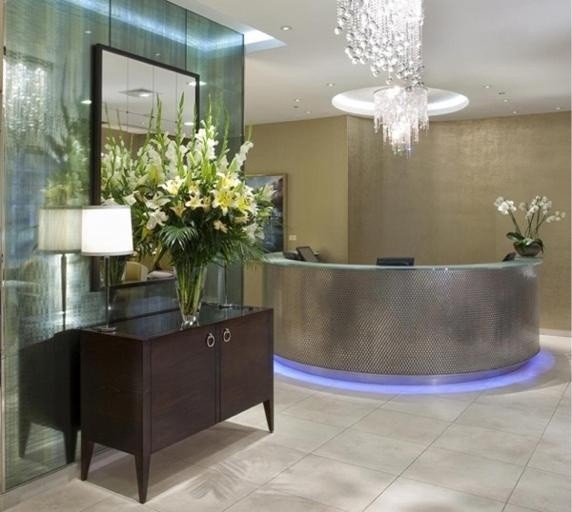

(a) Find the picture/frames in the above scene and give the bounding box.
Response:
[246,173,287,251]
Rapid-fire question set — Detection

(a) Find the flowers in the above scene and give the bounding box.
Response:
[142,93,281,314]
[495,195,566,253]
[44,125,89,207]
[103,102,186,286]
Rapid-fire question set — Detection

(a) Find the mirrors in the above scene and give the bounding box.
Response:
[91,44,199,290]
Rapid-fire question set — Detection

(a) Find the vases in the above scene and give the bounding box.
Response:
[512,241,541,258]
[171,260,207,324]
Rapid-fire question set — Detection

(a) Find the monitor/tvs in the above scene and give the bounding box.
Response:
[296,246,319,262]
[376,257,415,265]
[501,252,516,262]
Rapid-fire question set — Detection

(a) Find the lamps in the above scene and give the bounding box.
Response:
[374,86,427,154]
[38,207,83,310]
[335,0,423,91]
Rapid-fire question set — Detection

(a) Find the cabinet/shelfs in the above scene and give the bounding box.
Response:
[80,302,274,504]
[19,296,181,464]
